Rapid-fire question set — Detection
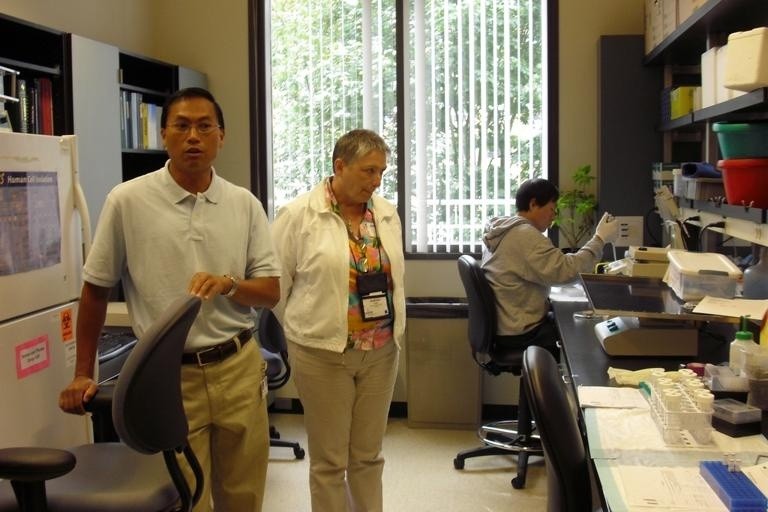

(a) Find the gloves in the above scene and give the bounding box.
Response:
[606,366,681,387]
[595,211,621,245]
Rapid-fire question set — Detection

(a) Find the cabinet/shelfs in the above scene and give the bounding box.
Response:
[0,12,210,264]
[638,1,767,266]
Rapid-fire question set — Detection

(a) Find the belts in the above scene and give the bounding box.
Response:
[180,329,252,366]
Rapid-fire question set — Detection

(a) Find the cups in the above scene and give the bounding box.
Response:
[744,347,768,412]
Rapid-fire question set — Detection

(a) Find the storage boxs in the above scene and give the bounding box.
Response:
[664,249,744,304]
[640,0,767,122]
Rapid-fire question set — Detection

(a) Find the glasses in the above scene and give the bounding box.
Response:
[357,238,369,273]
[167,121,221,134]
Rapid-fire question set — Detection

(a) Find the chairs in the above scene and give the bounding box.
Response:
[520,342,610,511]
[248,303,307,462]
[447,249,565,491]
[1,291,211,511]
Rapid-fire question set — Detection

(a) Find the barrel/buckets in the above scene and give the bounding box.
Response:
[711,121,767,161]
[714,156,768,211]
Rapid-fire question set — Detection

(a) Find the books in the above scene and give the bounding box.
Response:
[120,89,163,153]
[0,64,55,137]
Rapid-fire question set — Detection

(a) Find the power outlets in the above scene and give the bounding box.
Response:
[717,214,730,235]
[693,209,703,226]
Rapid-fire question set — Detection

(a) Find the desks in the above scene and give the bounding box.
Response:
[535,265,768,512]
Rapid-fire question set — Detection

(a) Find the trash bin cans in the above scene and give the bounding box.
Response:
[404,296,484,431]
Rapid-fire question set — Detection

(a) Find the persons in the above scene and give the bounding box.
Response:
[480,178,621,367]
[59,87,281,511]
[270,129,407,512]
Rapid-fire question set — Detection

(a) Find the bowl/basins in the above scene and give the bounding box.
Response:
[712,119,768,212]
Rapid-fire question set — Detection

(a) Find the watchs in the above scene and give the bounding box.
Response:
[221,274,241,299]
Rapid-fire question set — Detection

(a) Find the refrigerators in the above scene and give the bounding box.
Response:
[0,130,101,455]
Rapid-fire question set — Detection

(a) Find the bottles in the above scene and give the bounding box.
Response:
[743,247,768,300]
[651,368,715,445]
[728,315,758,376]
